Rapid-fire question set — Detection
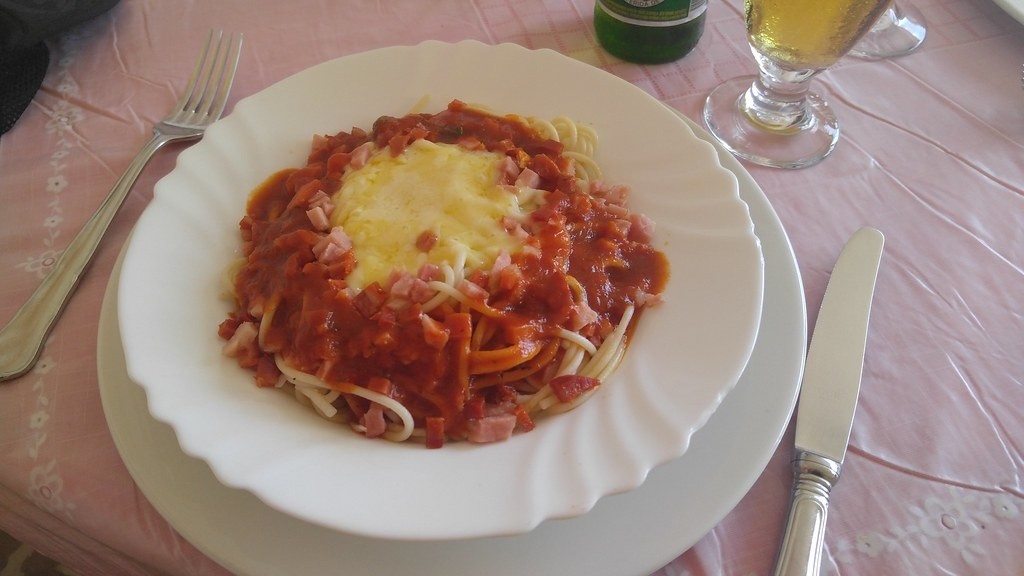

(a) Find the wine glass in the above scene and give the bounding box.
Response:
[703,2,927,170]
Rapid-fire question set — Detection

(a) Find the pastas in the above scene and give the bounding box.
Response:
[254,92,638,442]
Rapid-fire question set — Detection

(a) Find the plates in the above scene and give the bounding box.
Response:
[94,40,809,576]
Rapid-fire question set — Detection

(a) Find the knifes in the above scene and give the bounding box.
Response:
[773,225,885,575]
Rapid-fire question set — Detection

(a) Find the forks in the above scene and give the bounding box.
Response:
[0,27,244,381]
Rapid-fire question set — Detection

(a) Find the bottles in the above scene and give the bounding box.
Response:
[594,1,708,63]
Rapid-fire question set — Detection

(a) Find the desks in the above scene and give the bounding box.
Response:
[0,0,1024,576]
